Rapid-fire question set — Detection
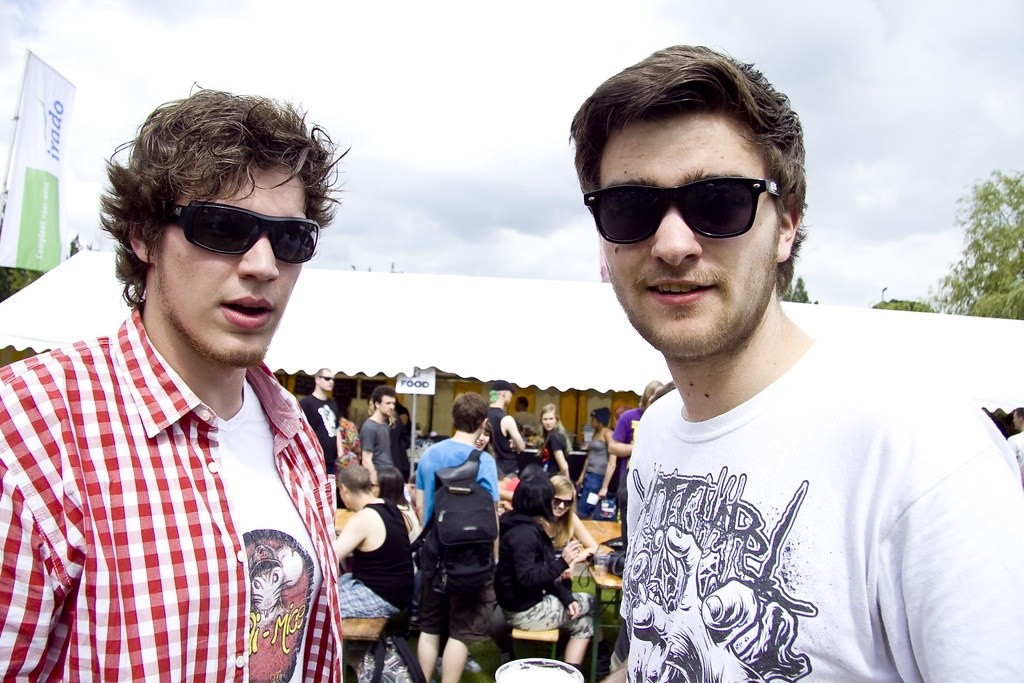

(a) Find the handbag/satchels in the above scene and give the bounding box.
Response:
[358,636,426,683]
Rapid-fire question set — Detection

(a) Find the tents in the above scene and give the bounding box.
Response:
[0,250,1024,396]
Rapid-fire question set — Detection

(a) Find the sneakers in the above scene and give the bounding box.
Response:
[501,651,510,664]
[435,657,443,673]
[464,655,482,673]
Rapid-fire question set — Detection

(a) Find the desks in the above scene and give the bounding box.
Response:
[572,519,623,683]
[335,508,354,529]
[522,448,588,481]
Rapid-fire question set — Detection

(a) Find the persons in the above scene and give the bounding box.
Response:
[539,403,573,479]
[1008,407,1024,485]
[487,380,526,478]
[576,407,617,522]
[512,397,540,428]
[414,391,499,683]
[333,464,422,619]
[0,89,343,683]
[499,465,614,674]
[361,385,412,473]
[608,380,664,482]
[298,369,343,475]
[569,46,1024,683]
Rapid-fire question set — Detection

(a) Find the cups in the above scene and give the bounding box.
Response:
[495,658,585,683]
[593,554,610,576]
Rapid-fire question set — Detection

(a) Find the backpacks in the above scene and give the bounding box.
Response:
[411,449,498,598]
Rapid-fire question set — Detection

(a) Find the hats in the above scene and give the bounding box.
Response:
[492,380,516,393]
[590,408,610,426]
[614,406,631,418]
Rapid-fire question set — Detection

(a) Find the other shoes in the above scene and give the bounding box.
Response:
[596,655,610,680]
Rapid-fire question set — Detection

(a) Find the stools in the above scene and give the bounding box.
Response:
[511,628,560,660]
[341,617,389,641]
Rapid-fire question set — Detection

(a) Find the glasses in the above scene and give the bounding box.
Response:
[552,497,573,508]
[583,178,781,245]
[317,374,335,381]
[165,201,320,265]
